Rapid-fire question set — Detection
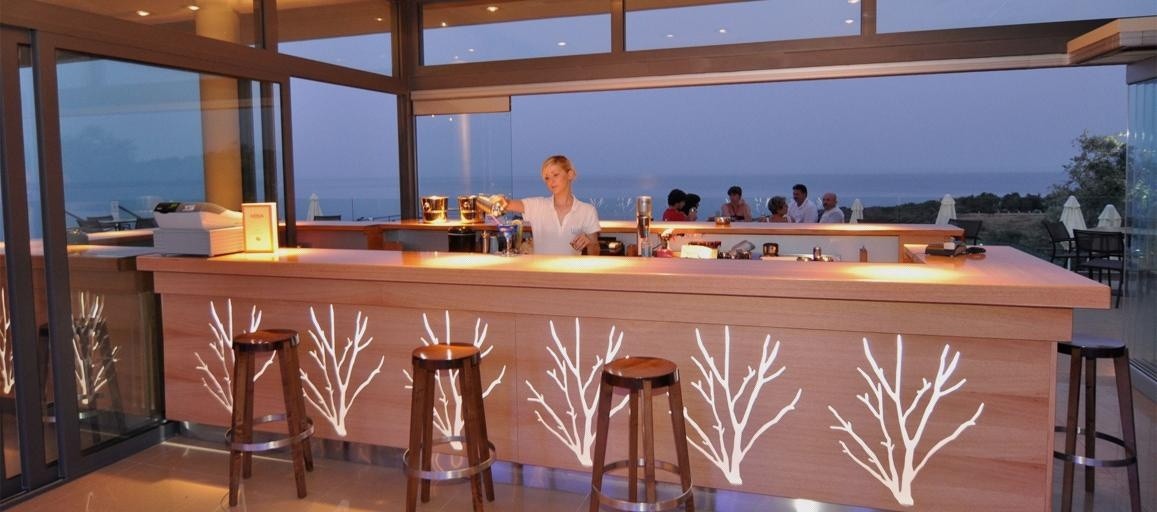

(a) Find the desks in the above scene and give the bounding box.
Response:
[1083,228,1156,296]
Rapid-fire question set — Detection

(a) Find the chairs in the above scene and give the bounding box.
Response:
[1072,227,1110,288]
[1040,220,1078,269]
[946,219,984,247]
[1087,232,1129,309]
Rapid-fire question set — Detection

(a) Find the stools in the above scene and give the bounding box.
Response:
[223,325,315,506]
[586,356,696,512]
[402,341,498,512]
[40,317,126,449]
[1051,331,1142,512]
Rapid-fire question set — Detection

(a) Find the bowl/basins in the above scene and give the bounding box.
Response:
[714,217,732,225]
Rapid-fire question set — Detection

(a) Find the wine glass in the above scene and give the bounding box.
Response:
[499,217,514,256]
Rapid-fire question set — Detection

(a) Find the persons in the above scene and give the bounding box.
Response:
[784,184,819,223]
[759,197,795,222]
[721,187,753,222]
[679,193,700,217]
[489,156,601,256]
[663,189,696,221]
[818,192,845,223]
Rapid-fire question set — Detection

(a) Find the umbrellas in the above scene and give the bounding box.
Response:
[850,198,864,224]
[1090,204,1127,274]
[1058,195,1088,272]
[935,194,957,225]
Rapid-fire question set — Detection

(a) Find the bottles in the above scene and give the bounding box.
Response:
[812,246,821,260]
[860,246,867,262]
[475,195,504,217]
